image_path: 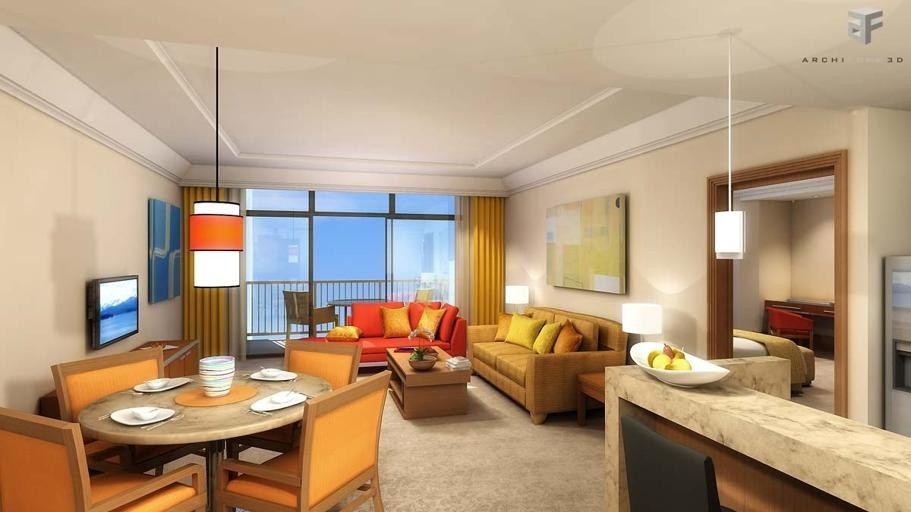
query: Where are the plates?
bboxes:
[133,377,192,392]
[251,370,298,381]
[111,407,175,425]
[250,390,307,412]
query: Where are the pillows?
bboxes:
[326,301,459,342]
[495,308,600,354]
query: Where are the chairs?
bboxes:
[414,288,434,304]
[621,416,737,512]
[225,340,364,512]
[282,290,339,342]
[51,345,210,508]
[767,307,815,351]
[216,369,393,512]
[0,407,207,512]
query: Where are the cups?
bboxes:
[200,355,235,397]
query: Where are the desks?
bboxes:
[327,299,391,326]
[767,298,834,335]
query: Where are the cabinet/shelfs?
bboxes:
[163,339,203,379]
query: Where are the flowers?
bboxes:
[408,327,435,360]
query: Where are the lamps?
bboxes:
[621,303,663,342]
[188,47,244,288]
[286,207,300,264]
[715,32,747,260]
[505,285,529,313]
[420,273,435,289]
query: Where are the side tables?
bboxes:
[576,370,605,426]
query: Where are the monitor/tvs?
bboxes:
[85,276,141,351]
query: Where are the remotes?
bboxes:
[399,346,415,351]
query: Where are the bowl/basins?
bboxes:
[273,393,294,403]
[145,378,169,389]
[129,407,159,419]
[631,340,731,388]
[261,368,281,377]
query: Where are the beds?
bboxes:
[732,336,815,386]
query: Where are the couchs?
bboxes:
[466,306,629,425]
[300,317,467,367]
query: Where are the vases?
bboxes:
[409,355,437,371]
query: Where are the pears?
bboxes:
[648,343,691,371]
